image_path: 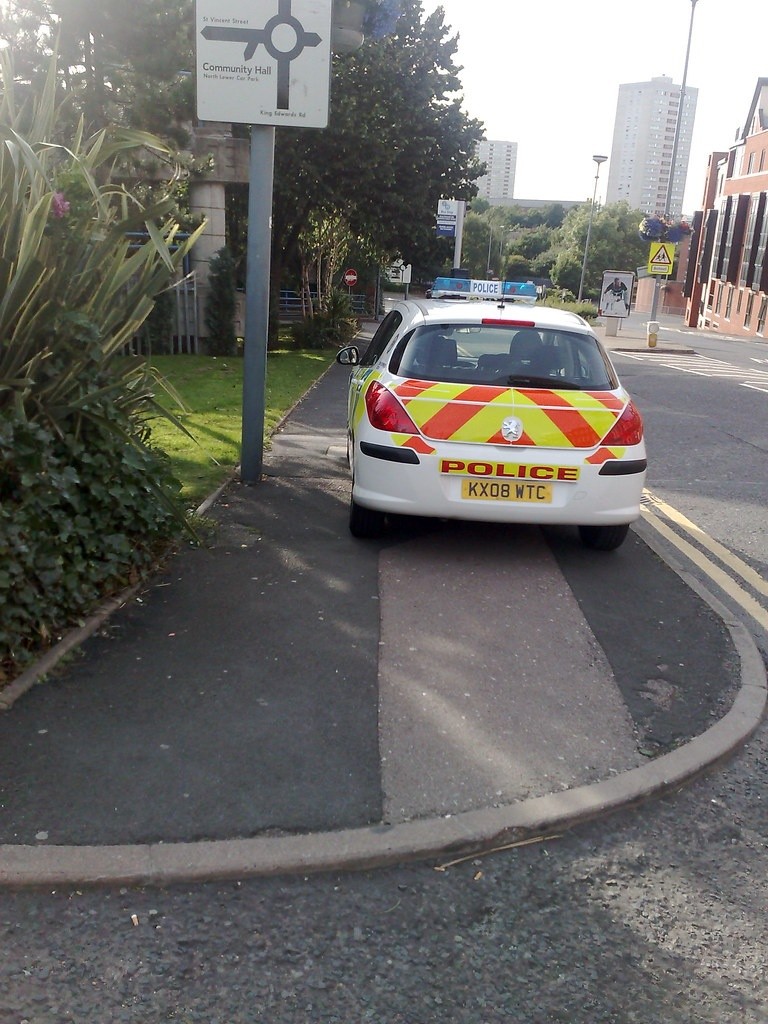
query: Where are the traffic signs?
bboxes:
[196,1,330,130]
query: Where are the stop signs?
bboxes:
[343,268,357,286]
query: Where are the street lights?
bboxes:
[578,155,608,303]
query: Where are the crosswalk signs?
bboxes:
[650,245,671,264]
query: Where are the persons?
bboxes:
[602,277,631,316]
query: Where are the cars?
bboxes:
[336,277,647,549]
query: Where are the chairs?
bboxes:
[417,336,457,367]
[530,345,567,375]
